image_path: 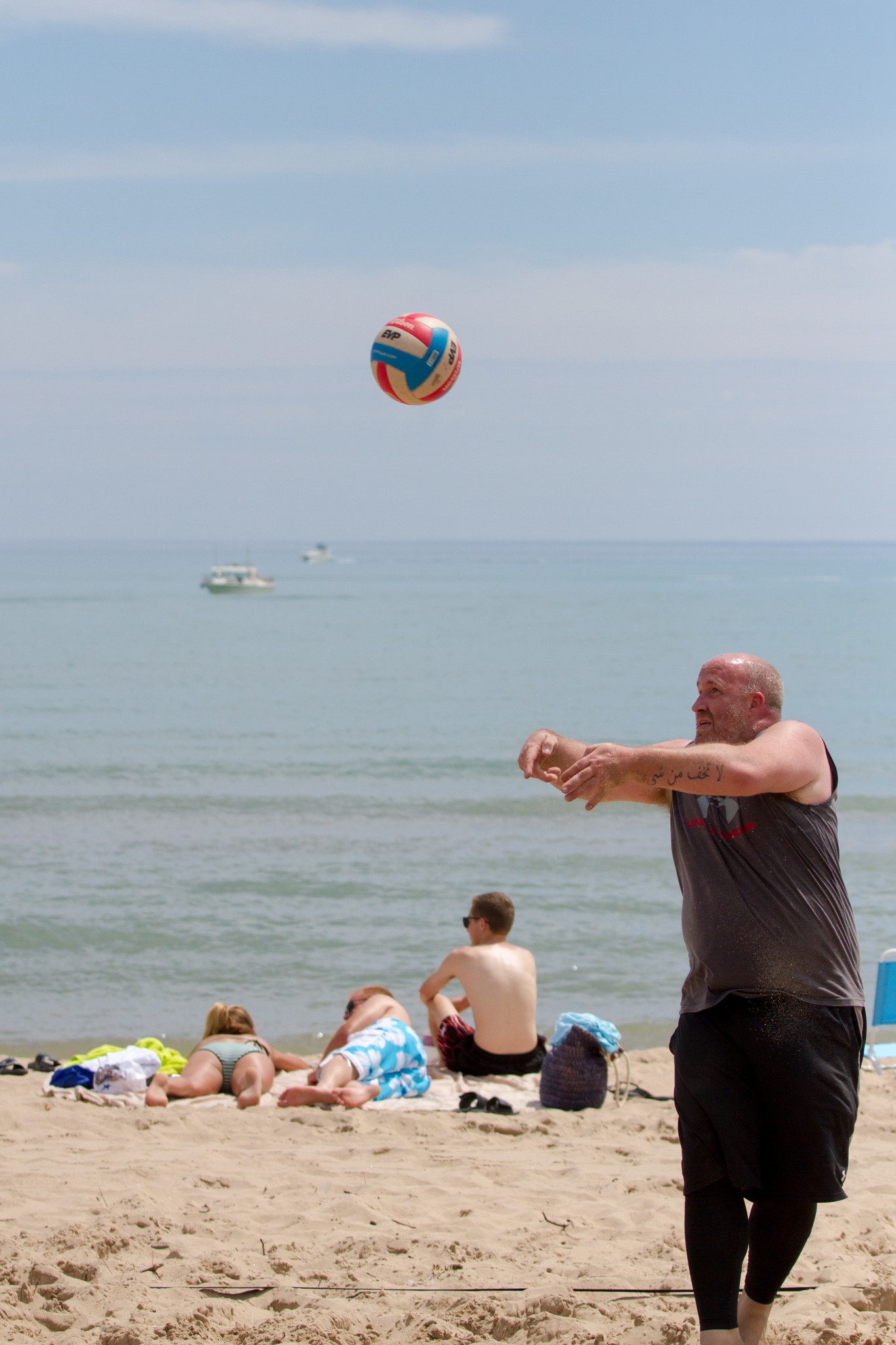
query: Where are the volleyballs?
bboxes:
[371,313,461,405]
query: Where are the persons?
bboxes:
[277,986,432,1110]
[518,652,868,1345]
[420,891,548,1076]
[144,1002,314,1110]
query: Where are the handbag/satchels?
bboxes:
[539,1021,631,1110]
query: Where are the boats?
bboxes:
[200,564,278,594]
[302,537,333,562]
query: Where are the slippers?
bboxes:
[28,1053,61,1072]
[0,1057,27,1074]
[452,1091,520,1116]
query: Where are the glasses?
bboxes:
[344,998,368,1015]
[462,917,490,928]
